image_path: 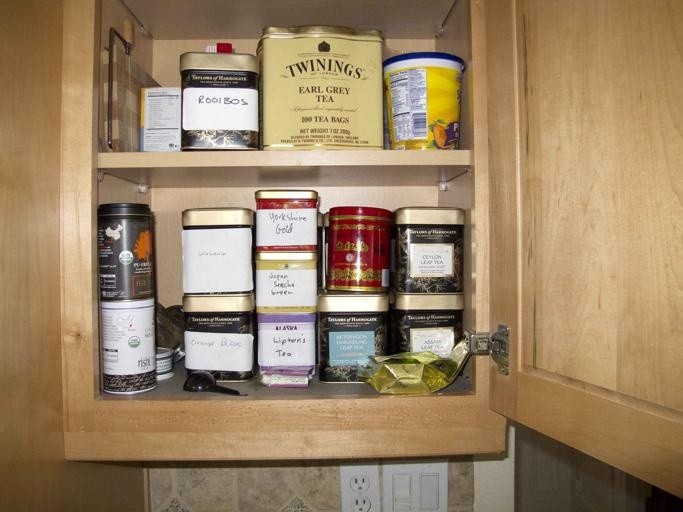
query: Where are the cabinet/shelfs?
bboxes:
[60,1,683,501]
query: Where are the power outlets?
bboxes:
[339,465,381,512]
